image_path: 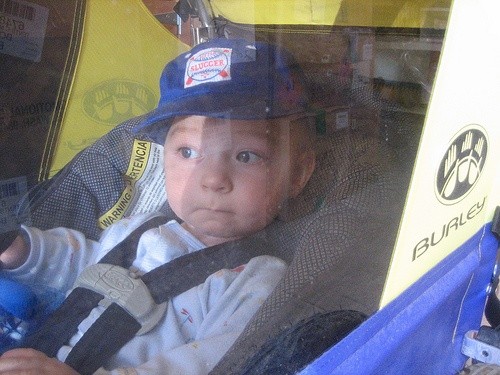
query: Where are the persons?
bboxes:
[0,37,316,375]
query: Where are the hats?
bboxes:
[133,38,316,137]
